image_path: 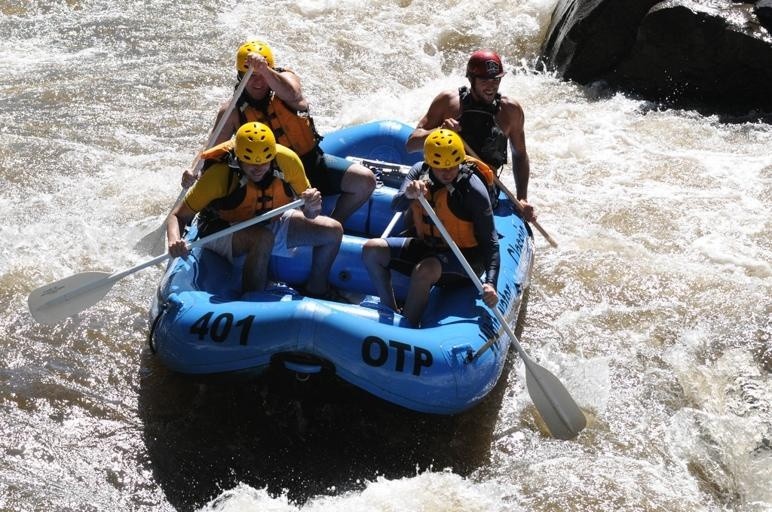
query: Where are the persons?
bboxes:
[180,41,376,223]
[167,121,351,303]
[361,126,500,326]
[405,51,533,217]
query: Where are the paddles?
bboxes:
[29,188,318,328]
[417,192,587,440]
[134,63,254,257]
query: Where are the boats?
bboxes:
[148,119,534,419]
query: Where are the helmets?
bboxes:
[423,129,467,169]
[466,50,507,80]
[234,122,277,165]
[235,41,275,73]
